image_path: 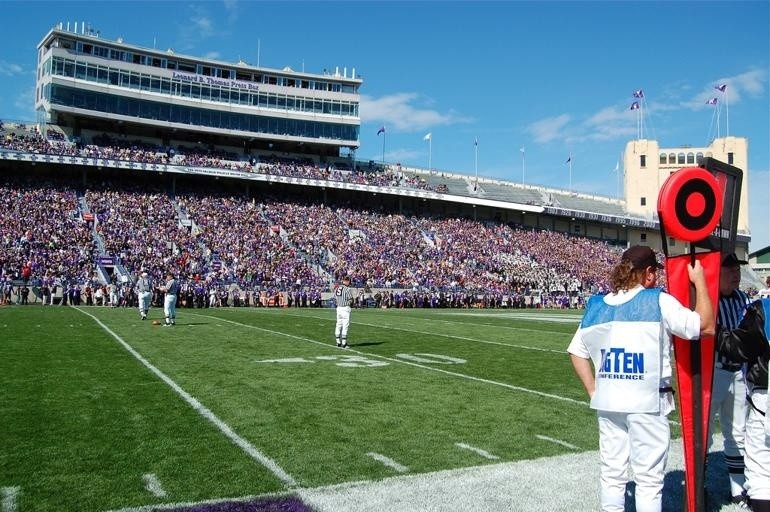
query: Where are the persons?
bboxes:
[0,124,768,310]
[159,272,179,327]
[134,272,154,320]
[566,246,716,512]
[716,298,770,512]
[333,277,354,349]
[703,253,753,510]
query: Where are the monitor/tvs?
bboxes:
[695,156,743,253]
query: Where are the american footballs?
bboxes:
[151,319,162,325]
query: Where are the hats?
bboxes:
[722,253,748,267]
[622,245,664,269]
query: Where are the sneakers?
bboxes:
[141,314,146,320]
[729,490,751,512]
[163,321,175,327]
[336,343,351,348]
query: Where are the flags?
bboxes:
[630,89,643,109]
[705,97,717,104]
[715,85,726,92]
